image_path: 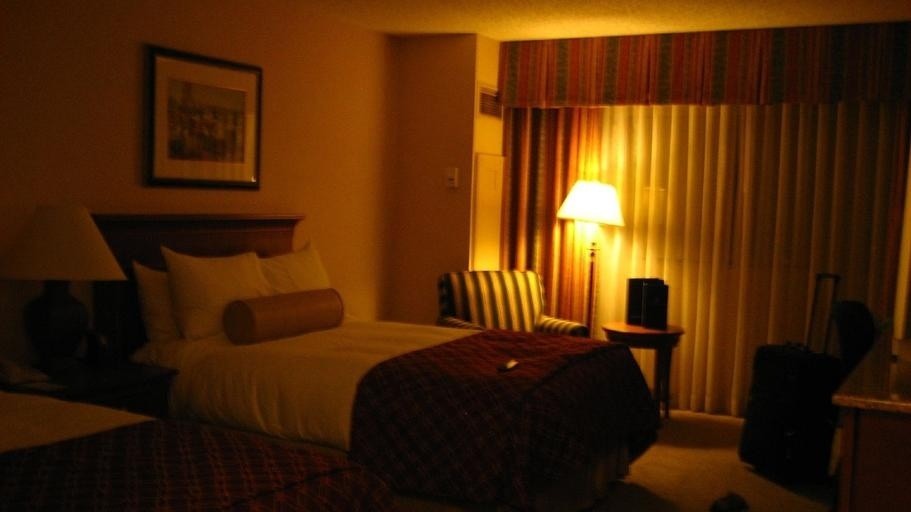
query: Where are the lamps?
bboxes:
[557,180,625,328]
[0,204,127,371]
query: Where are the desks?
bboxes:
[833,322,911,512]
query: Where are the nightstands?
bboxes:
[0,363,181,416]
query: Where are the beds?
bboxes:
[90,213,658,512]
[1,392,390,512]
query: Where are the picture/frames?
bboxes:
[140,43,263,191]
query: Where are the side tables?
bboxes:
[601,321,684,418]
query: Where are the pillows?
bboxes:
[161,246,272,342]
[223,288,344,345]
[134,261,181,341]
[259,237,331,293]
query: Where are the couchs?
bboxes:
[436,270,590,338]
